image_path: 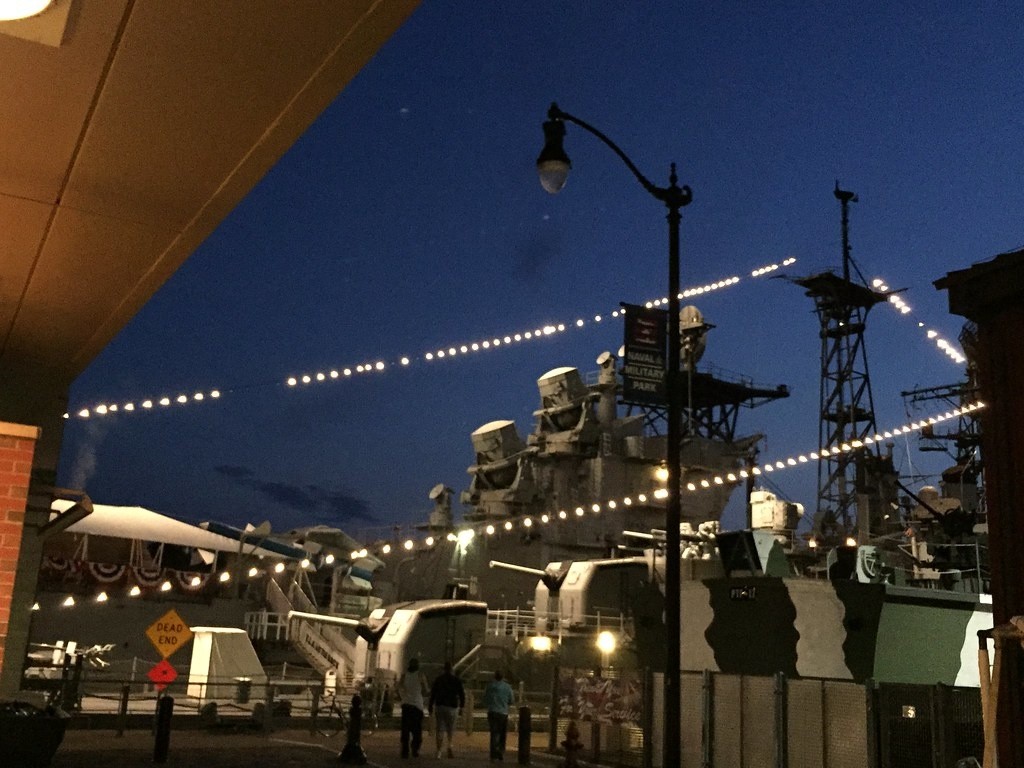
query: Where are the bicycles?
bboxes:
[312,689,380,740]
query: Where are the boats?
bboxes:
[18,499,401,697]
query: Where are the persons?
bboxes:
[429,661,465,759]
[398,658,430,759]
[483,670,515,763]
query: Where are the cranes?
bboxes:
[901,365,980,464]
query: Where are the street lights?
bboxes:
[535,95,699,768]
[530,633,563,756]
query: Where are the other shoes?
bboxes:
[490,754,497,760]
[436,752,440,759]
[400,751,408,759]
[448,746,455,758]
[499,756,503,760]
[412,752,421,757]
